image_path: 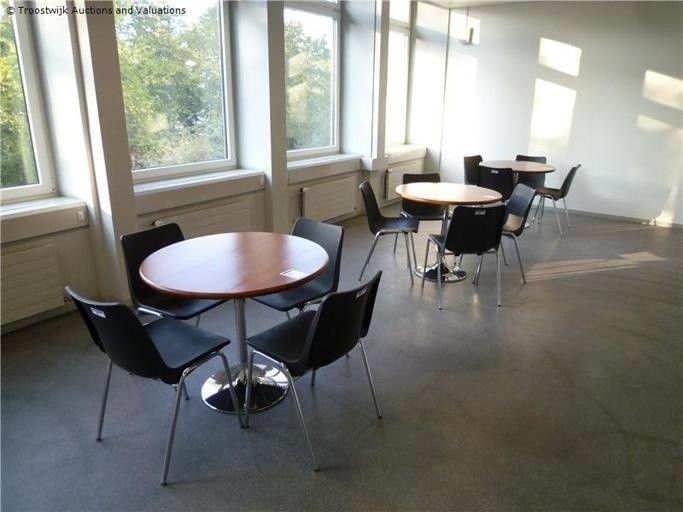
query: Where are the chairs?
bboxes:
[254,218,345,321]
[243,270,383,471]
[66,286,246,485]
[359,153,581,309]
[120,222,237,328]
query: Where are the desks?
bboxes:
[137,232,330,414]
